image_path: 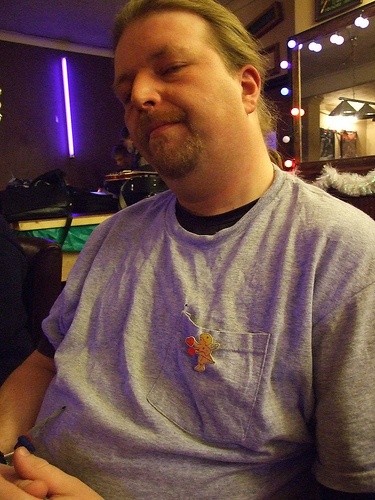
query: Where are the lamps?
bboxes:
[329,34,375,119]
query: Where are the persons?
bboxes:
[97,126,168,211]
[0,0,375,500]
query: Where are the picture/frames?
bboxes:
[244,2,283,38]
[315,0,363,21]
[258,42,281,78]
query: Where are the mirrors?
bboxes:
[287,2,375,176]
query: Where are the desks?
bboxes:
[8,211,119,282]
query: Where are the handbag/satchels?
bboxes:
[7,168,68,207]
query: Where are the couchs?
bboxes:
[15,236,61,346]
